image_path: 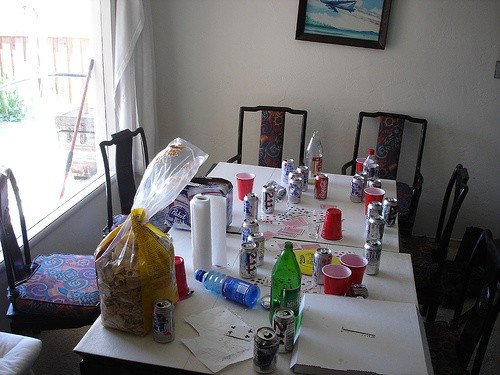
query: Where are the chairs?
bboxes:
[0,106,500,375]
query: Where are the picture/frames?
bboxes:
[294,0,393,50]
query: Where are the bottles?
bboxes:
[193,268,260,308]
[363,149,379,188]
[268,241,302,337]
[305,130,323,185]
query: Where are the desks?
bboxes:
[171,162,401,253]
[73,227,419,375]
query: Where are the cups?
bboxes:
[363,186,386,216]
[175,256,189,301]
[356,158,374,172]
[321,263,352,296]
[339,253,368,286]
[235,172,256,201]
[320,208,343,241]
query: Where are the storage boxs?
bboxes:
[290,293,434,375]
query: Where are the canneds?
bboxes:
[296,165,309,191]
[350,172,382,203]
[364,239,382,275]
[152,299,175,343]
[315,174,328,199]
[281,158,295,184]
[367,201,383,217]
[365,215,385,246]
[242,193,258,220]
[346,285,369,300]
[271,308,296,353]
[382,197,398,226]
[239,218,265,278]
[252,327,280,374]
[262,171,302,213]
[313,248,333,285]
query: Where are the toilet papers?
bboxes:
[189,193,227,272]
